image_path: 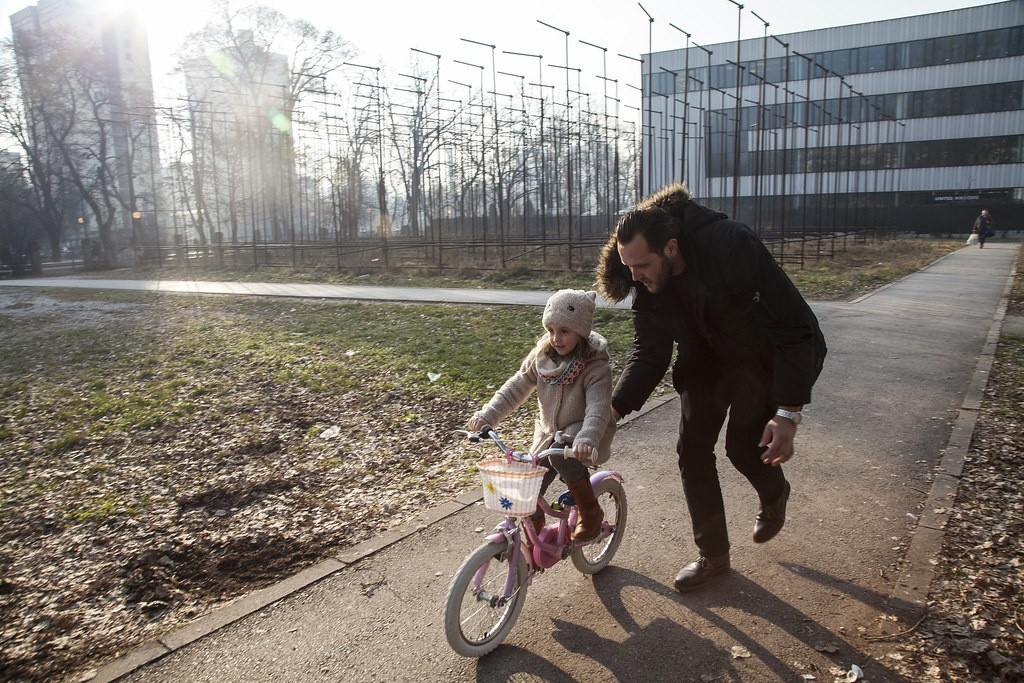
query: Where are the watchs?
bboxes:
[776,408,802,425]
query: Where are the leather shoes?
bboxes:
[752,479,791,544]
[672,553,730,590]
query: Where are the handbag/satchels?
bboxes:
[983,231,996,237]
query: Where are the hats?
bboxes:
[542,289,596,340]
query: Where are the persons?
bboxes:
[972,208,993,249]
[594,185,828,593]
[468,288,617,572]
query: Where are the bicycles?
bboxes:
[439,417,630,660]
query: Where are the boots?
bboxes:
[564,470,604,542]
[520,517,545,550]
